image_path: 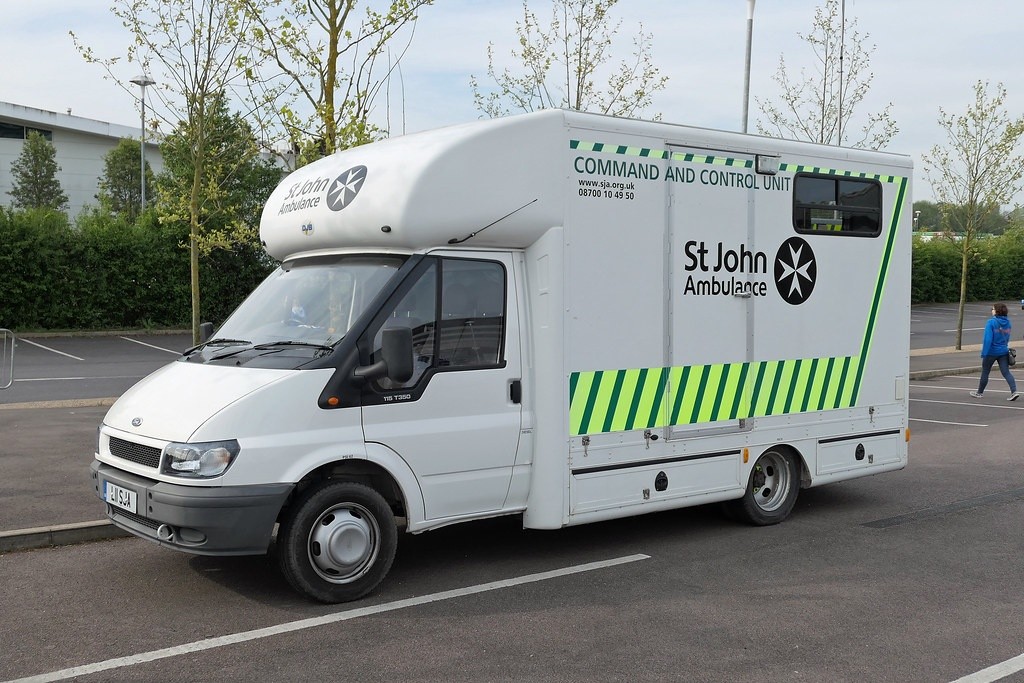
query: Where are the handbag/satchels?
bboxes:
[1008,347,1016,366]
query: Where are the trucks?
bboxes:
[91,108,913,606]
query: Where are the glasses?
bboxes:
[992,308,995,310]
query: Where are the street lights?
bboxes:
[128,75,157,212]
[915,211,922,230]
[914,218,917,230]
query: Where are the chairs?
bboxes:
[416,283,480,364]
[385,289,423,346]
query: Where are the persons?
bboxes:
[970,302,1019,401]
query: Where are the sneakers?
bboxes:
[1006,391,1019,401]
[970,391,984,398]
